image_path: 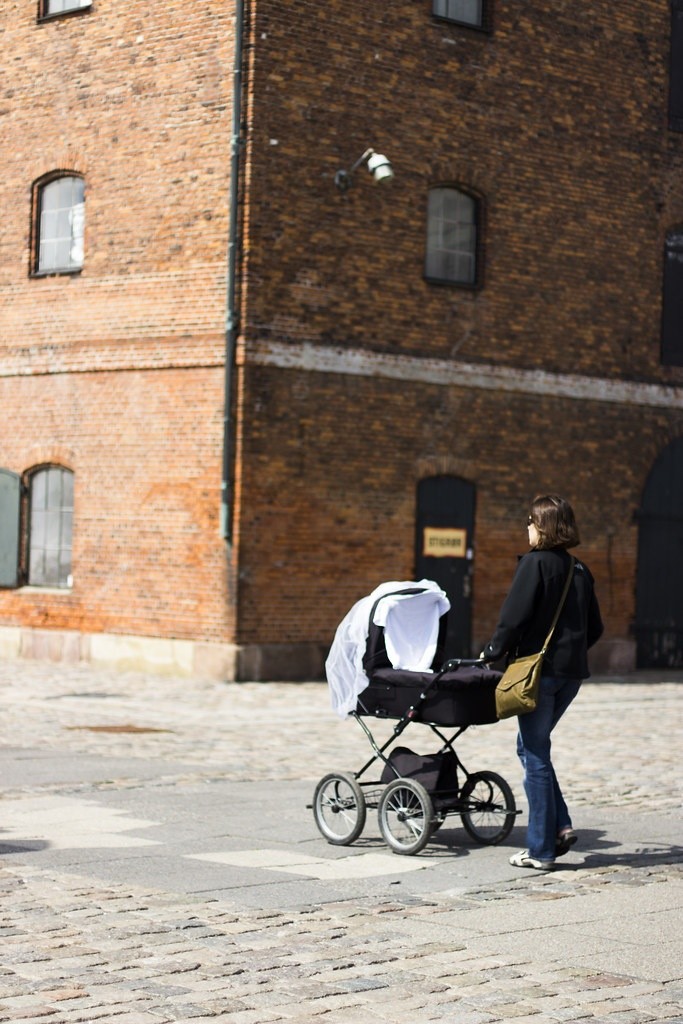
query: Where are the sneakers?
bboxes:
[508,848,554,870]
[552,823,579,857]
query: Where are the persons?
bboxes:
[480,496,604,868]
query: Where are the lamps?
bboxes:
[334,147,395,194]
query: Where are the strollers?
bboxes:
[311,578,523,855]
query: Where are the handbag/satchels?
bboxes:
[381,745,458,799]
[494,650,543,717]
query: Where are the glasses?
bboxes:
[526,515,534,523]
